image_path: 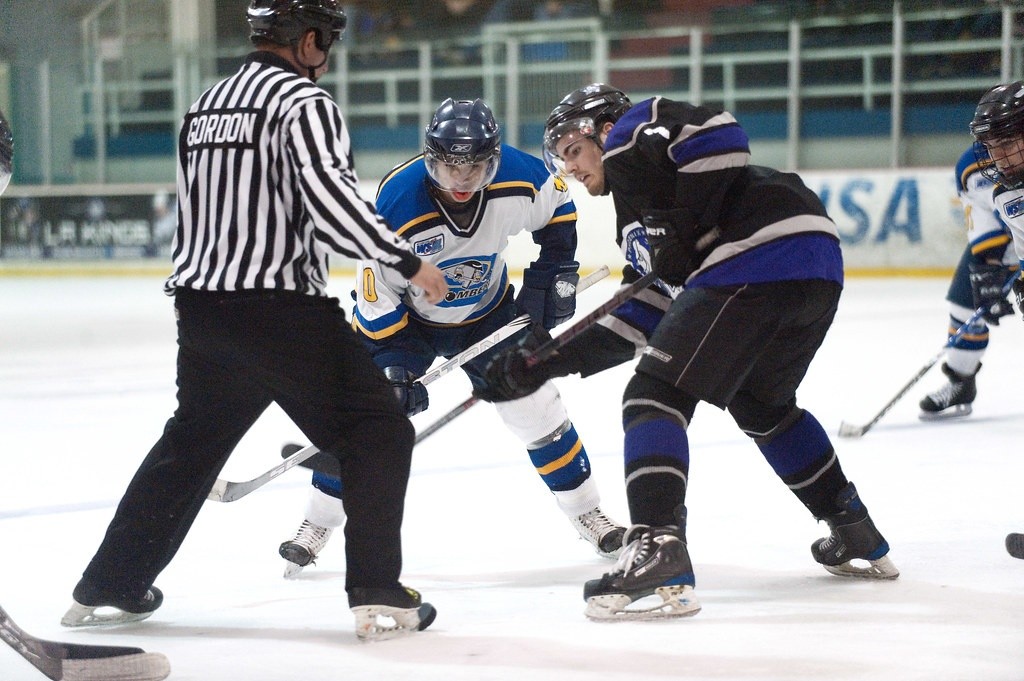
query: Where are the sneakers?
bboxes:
[573,506,630,558]
[348,588,436,638]
[812,481,900,578]
[279,520,332,577]
[60,578,164,626]
[584,504,701,618]
[917,363,982,419]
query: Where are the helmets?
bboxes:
[245,0,346,52]
[424,98,501,164]
[545,84,633,138]
[969,81,1024,142]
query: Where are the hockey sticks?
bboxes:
[203,263,614,503]
[1004,532,1024,560]
[281,269,661,474]
[0,604,172,681]
[836,269,1024,440]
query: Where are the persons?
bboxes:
[919,81,1024,421]
[59,0,436,640]
[478,82,900,621]
[339,0,1024,108]
[279,98,628,580]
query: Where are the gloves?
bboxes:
[485,323,568,401]
[641,208,699,286]
[513,261,580,330]
[384,366,429,418]
[967,260,1014,325]
[1012,278,1024,313]
